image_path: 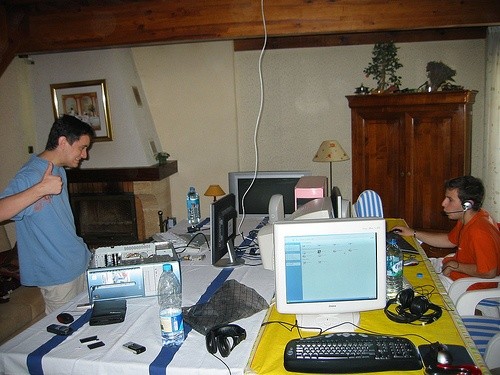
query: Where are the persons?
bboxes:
[392,175,500,315]
[0,115,94,314]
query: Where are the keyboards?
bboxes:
[284,333,422,374]
[153,231,197,250]
[384,232,419,254]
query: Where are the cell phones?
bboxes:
[47,324,73,336]
[122,341,146,354]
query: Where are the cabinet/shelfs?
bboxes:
[346,93,478,257]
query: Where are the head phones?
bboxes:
[382,288,443,326]
[206,324,246,357]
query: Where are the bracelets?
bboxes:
[414,230,416,238]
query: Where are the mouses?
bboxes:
[57,313,75,324]
[403,258,419,266]
[429,341,452,367]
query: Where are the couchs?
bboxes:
[0,220,49,344]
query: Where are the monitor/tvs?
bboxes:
[272,217,386,333]
[210,193,245,268]
[228,171,305,218]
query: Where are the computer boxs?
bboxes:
[294,176,328,211]
[85,242,182,304]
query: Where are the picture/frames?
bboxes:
[50,79,112,142]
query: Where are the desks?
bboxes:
[0,215,493,375]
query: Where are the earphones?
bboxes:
[463,202,472,210]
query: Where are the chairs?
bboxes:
[444,253,500,305]
[456,289,500,369]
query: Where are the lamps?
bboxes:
[204,185,226,202]
[312,140,350,196]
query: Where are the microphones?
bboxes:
[440,209,466,216]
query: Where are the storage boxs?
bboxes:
[87,242,181,303]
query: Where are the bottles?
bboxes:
[157,264,185,346]
[187,187,200,225]
[386,238,403,302]
